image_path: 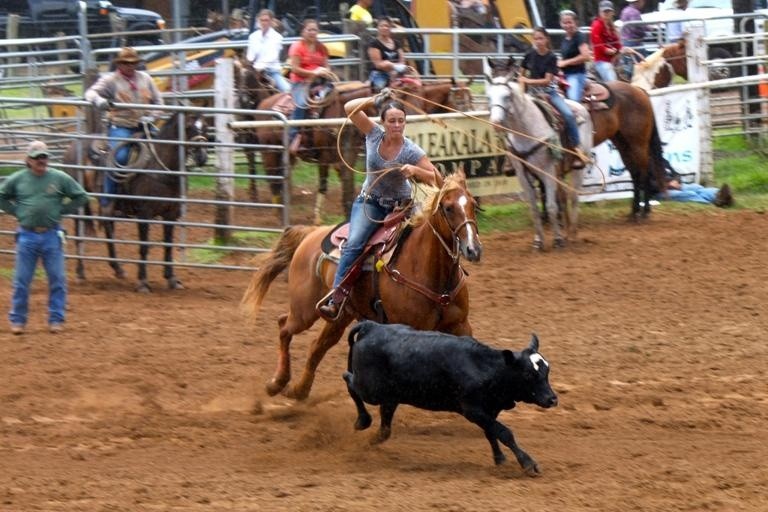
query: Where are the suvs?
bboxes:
[616,1,768,87]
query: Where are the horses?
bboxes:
[240,163,483,401]
[630,38,687,90]
[231,53,274,204]
[482,72,595,254]
[256,74,423,226]
[341,77,475,114]
[64,110,208,295]
[487,55,670,227]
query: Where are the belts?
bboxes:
[20,225,50,233]
[361,191,411,210]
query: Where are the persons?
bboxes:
[319,88,437,315]
[83,44,168,227]
[521,27,595,167]
[239,1,691,156]
[1,137,93,339]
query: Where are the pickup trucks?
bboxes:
[0,0,166,81]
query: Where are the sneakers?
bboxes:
[11,323,65,334]
[570,156,585,169]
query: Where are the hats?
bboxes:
[600,1,613,12]
[27,141,52,160]
[112,47,142,64]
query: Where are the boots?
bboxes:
[320,299,344,320]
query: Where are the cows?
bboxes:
[342,319,558,478]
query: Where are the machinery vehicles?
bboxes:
[39,1,563,153]
